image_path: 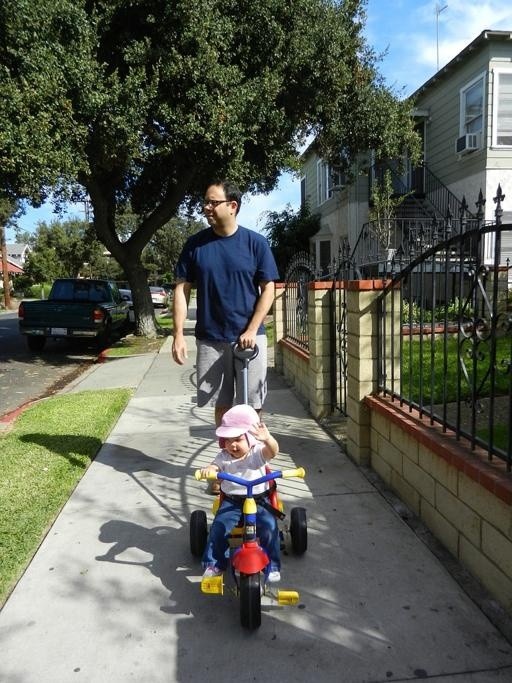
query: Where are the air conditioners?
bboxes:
[455,133,478,156]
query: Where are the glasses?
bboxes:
[203,200,231,206]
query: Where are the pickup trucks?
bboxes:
[17,277,131,357]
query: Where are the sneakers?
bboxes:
[268,571,281,583]
[203,566,219,578]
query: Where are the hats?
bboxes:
[215,404,260,447]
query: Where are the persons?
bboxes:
[198,404,286,581]
[171,179,278,443]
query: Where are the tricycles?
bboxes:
[189,465,308,635]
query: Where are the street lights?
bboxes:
[435,1,449,71]
[83,262,91,278]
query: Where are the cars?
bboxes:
[118,288,133,307]
[149,286,170,308]
